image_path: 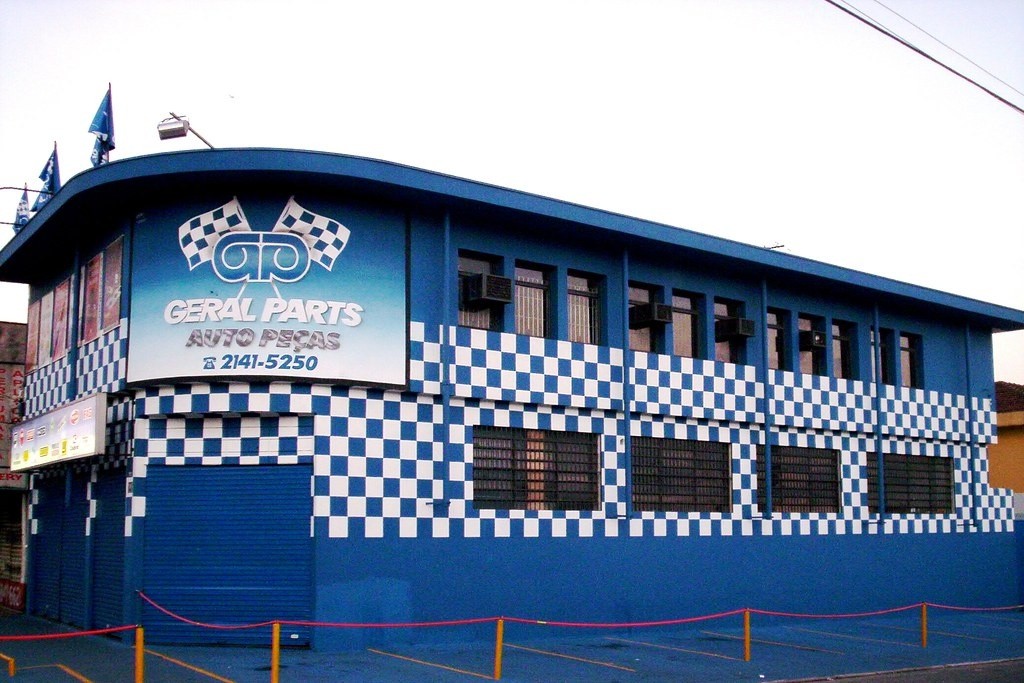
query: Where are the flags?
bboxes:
[30,149,61,213]
[88,89,116,165]
[13,187,30,235]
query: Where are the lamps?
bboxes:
[157,120,189,140]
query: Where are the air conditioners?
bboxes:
[463,273,513,307]
[801,330,827,351]
[637,303,672,324]
[725,317,755,337]
